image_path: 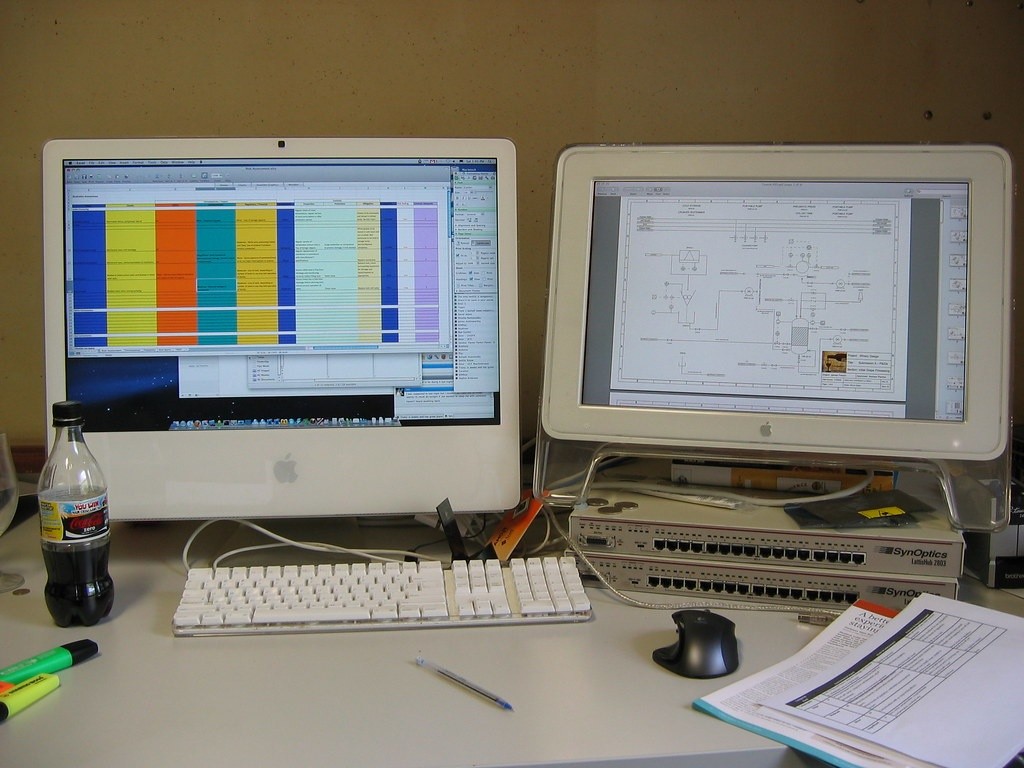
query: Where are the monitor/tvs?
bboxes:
[534,146,1013,527]
[38,137,520,565]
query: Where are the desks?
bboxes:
[0,473,1024,768]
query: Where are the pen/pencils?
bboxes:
[413,653,515,713]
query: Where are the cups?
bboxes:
[0,433,20,536]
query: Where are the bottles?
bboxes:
[34,399,116,628]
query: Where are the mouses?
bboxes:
[650,609,738,680]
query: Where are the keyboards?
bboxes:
[171,556,592,636]
